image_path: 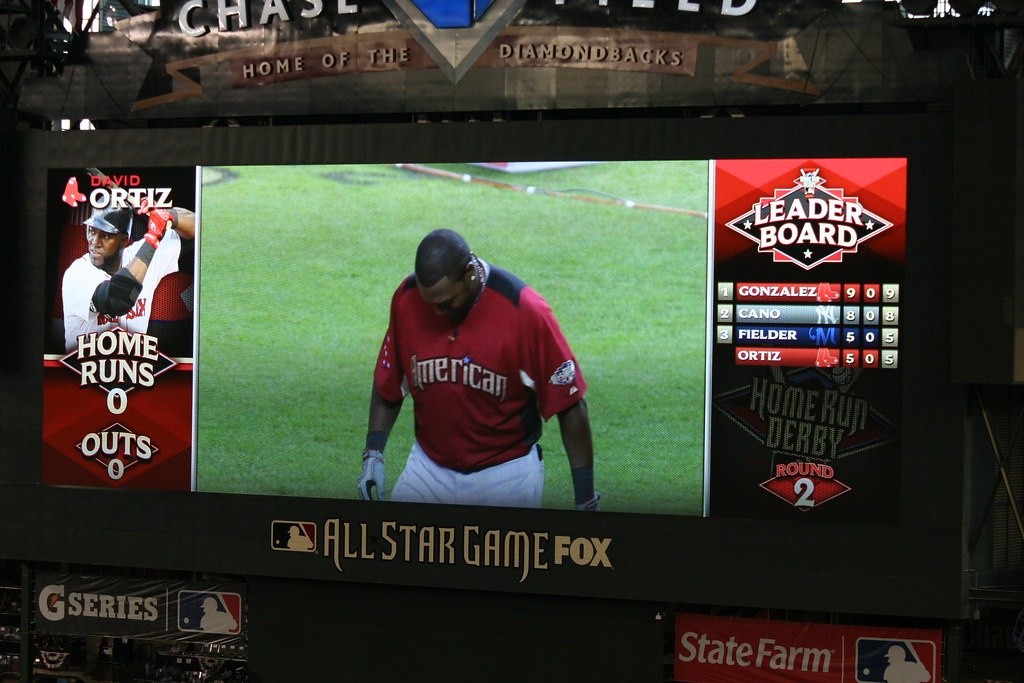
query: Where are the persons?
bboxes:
[62,195,196,355]
[357,229,601,511]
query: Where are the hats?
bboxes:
[83,193,133,234]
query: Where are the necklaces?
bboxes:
[472,254,485,301]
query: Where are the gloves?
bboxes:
[357,446,386,500]
[134,196,162,214]
[576,492,601,511]
[144,208,174,248]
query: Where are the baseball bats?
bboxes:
[87,168,172,230]
